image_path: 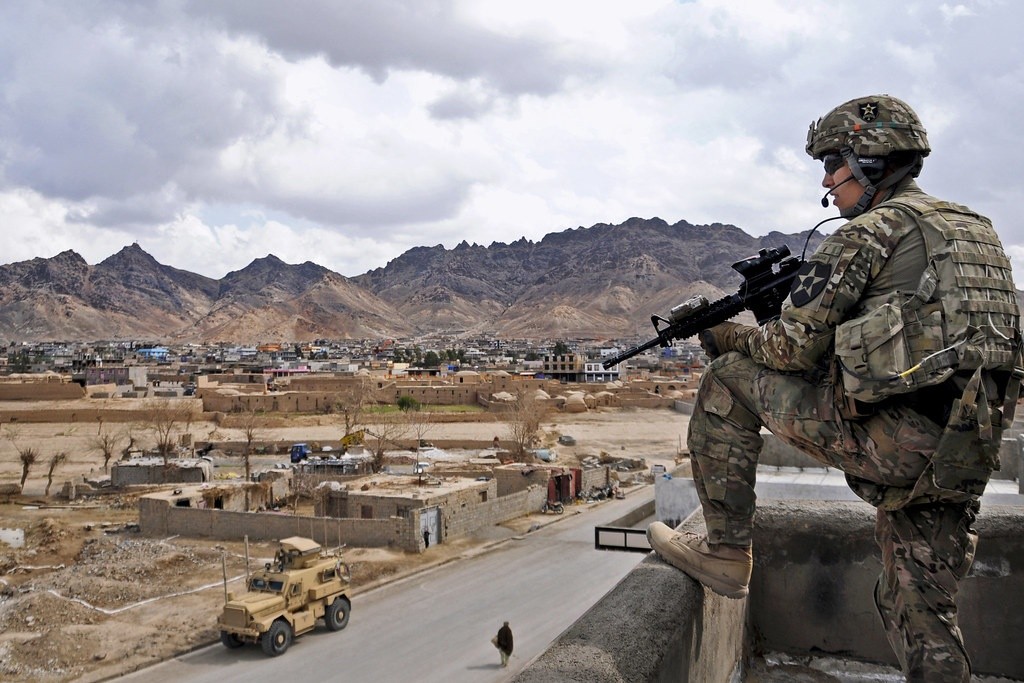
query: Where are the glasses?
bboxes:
[823,150,845,174]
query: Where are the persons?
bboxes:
[494,620,513,667]
[650,93,1024,683]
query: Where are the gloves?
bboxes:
[698,320,743,361]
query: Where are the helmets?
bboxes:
[807,97,931,159]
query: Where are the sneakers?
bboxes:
[645,522,753,599]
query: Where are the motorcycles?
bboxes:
[540,498,565,515]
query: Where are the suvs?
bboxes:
[217,536,353,657]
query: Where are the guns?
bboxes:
[600,243,809,371]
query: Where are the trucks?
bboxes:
[290,443,345,464]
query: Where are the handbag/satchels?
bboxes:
[834,303,916,402]
